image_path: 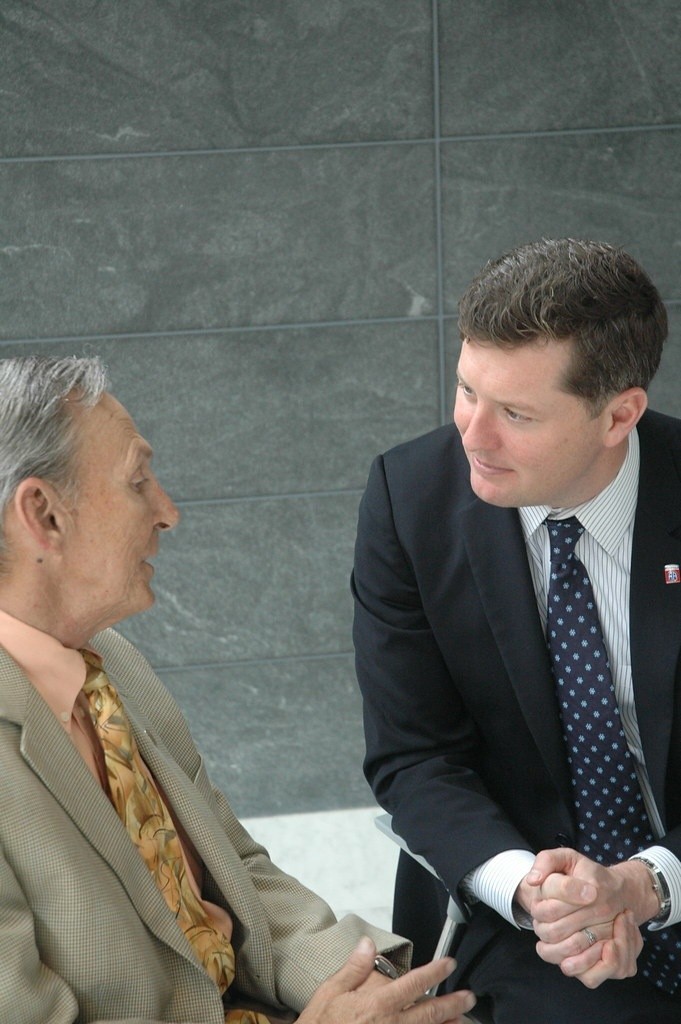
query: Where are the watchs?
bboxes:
[374,955,399,978]
[632,857,672,920]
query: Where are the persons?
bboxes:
[0,356,474,1024]
[350,239,681,1024]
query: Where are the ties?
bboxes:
[542,517,681,996]
[77,651,270,1024]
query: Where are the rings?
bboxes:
[583,929,596,945]
